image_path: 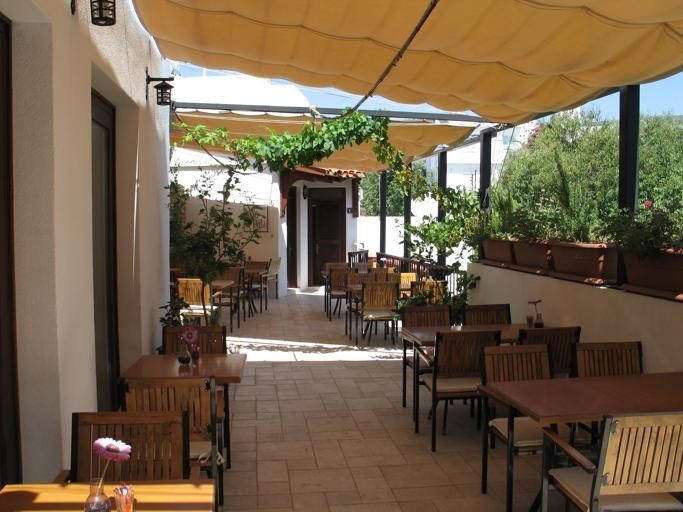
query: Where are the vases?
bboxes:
[84,477,112,512]
[533,313,544,327]
[622,248,683,294]
[178,342,190,363]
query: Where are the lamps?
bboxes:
[71,0,116,28]
[145,66,174,106]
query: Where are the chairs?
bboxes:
[118,374,222,504]
[71,410,190,480]
[462,303,511,325]
[518,325,581,383]
[411,281,447,304]
[478,343,594,512]
[571,342,643,453]
[423,329,501,452]
[314,245,417,345]
[172,256,283,330]
[402,305,450,327]
[541,412,683,510]
[161,325,226,355]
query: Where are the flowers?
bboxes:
[609,201,683,248]
[528,298,543,316]
[91,436,131,500]
[182,327,196,349]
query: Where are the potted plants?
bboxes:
[512,193,550,270]
[549,198,608,278]
[482,186,513,267]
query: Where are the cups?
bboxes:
[526,315,535,330]
[115,489,134,512]
[190,346,201,368]
